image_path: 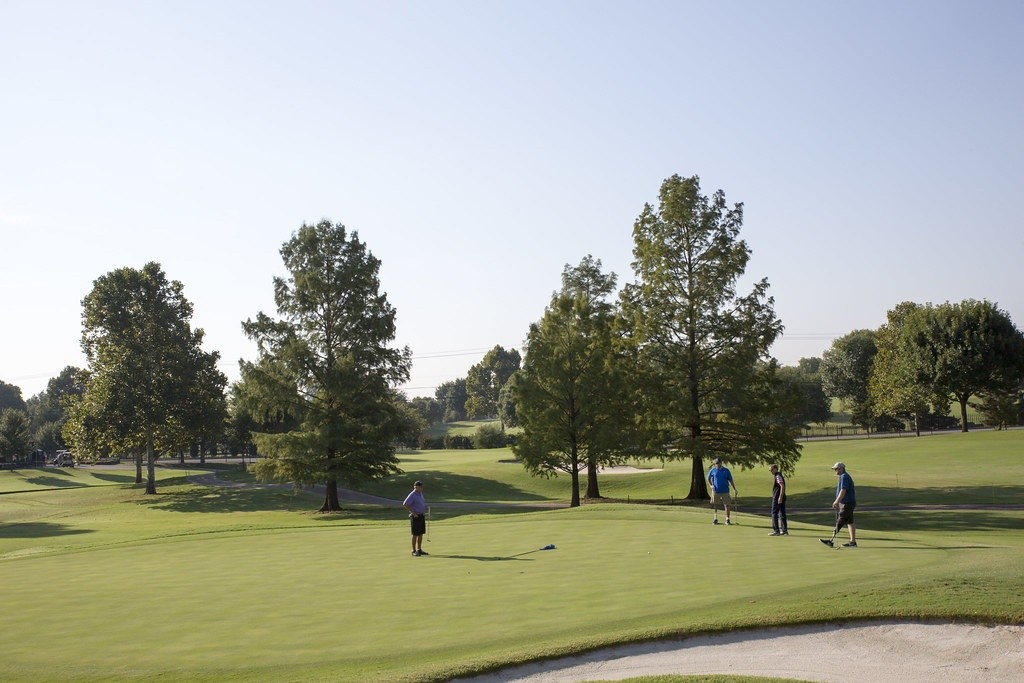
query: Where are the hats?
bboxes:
[831,462,846,469]
[715,457,722,464]
[413,481,423,486]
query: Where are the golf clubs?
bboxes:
[735,493,739,525]
[835,509,840,550]
[427,506,432,543]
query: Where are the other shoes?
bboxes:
[780,531,789,535]
[768,531,780,536]
[725,519,730,525]
[416,550,428,555]
[713,519,718,524]
[412,551,421,556]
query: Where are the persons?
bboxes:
[707,458,738,525]
[768,465,788,536]
[403,481,431,556]
[819,463,857,548]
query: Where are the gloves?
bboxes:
[733,489,738,495]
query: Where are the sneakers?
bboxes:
[842,541,857,547]
[819,538,833,547]
